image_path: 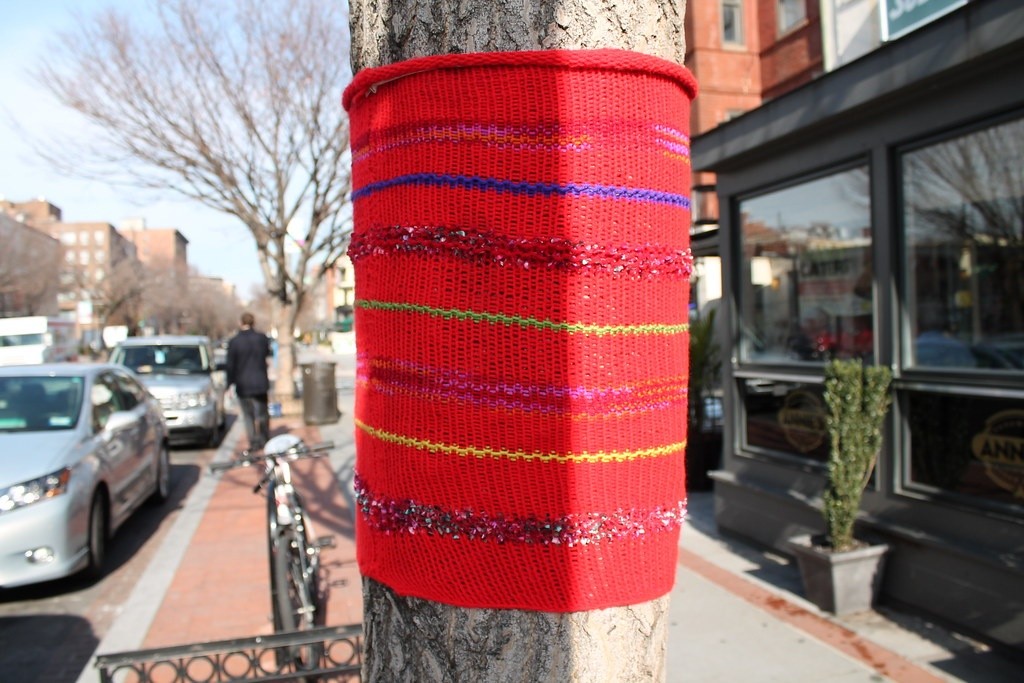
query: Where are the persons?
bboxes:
[225,313,274,448]
[916,302,975,367]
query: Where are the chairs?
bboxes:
[52,387,79,414]
[18,385,51,412]
[134,349,155,366]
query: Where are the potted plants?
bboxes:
[786,357,890,617]
[683,307,723,496]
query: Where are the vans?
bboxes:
[0,316,79,365]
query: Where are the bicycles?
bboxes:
[209,435,339,683]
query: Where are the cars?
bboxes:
[742,318,1024,506]
[0,364,170,588]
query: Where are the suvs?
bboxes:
[109,335,226,448]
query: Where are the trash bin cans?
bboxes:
[301,362,339,425]
